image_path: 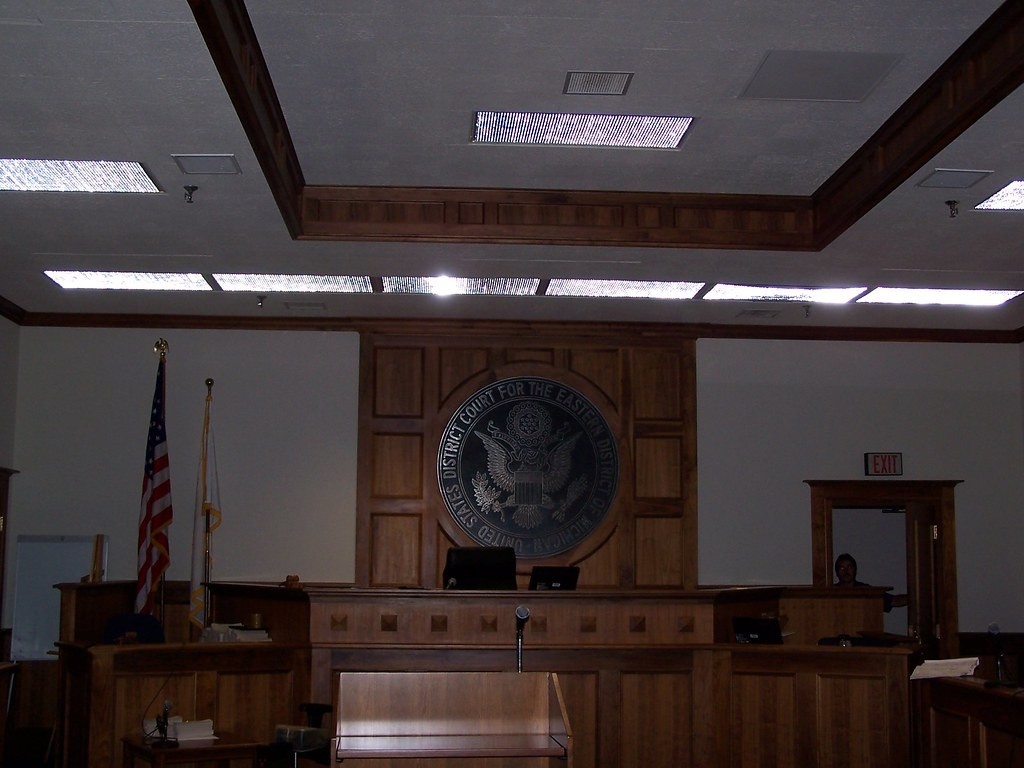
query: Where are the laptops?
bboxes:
[528,566,580,591]
[729,616,784,646]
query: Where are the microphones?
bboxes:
[516,606,530,634]
[445,578,456,589]
[988,622,1005,660]
[162,703,171,732]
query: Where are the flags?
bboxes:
[187,395,222,634]
[134,359,172,618]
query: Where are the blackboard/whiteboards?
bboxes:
[10,532,110,662]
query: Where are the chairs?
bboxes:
[443,547,521,592]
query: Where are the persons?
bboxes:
[832,554,908,622]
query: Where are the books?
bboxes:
[909,655,980,681]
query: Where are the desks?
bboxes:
[122,728,264,768]
